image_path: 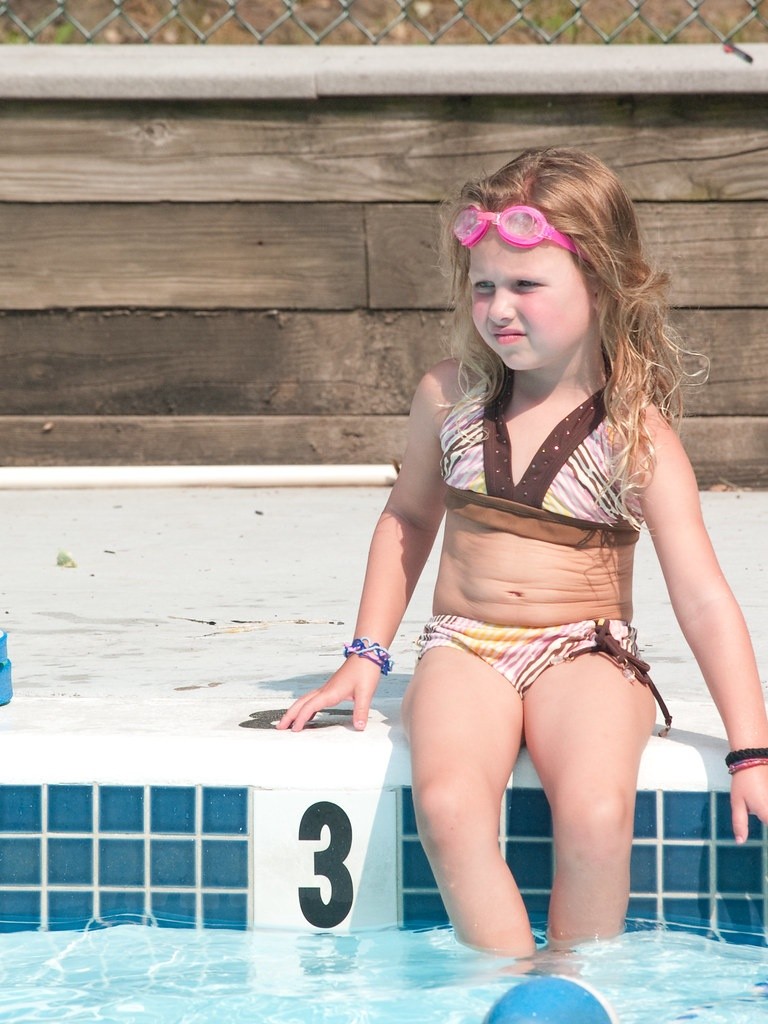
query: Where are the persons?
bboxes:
[275,143,768,938]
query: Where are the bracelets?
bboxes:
[343,637,394,675]
[725,748,768,774]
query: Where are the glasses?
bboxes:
[453,203,584,253]
